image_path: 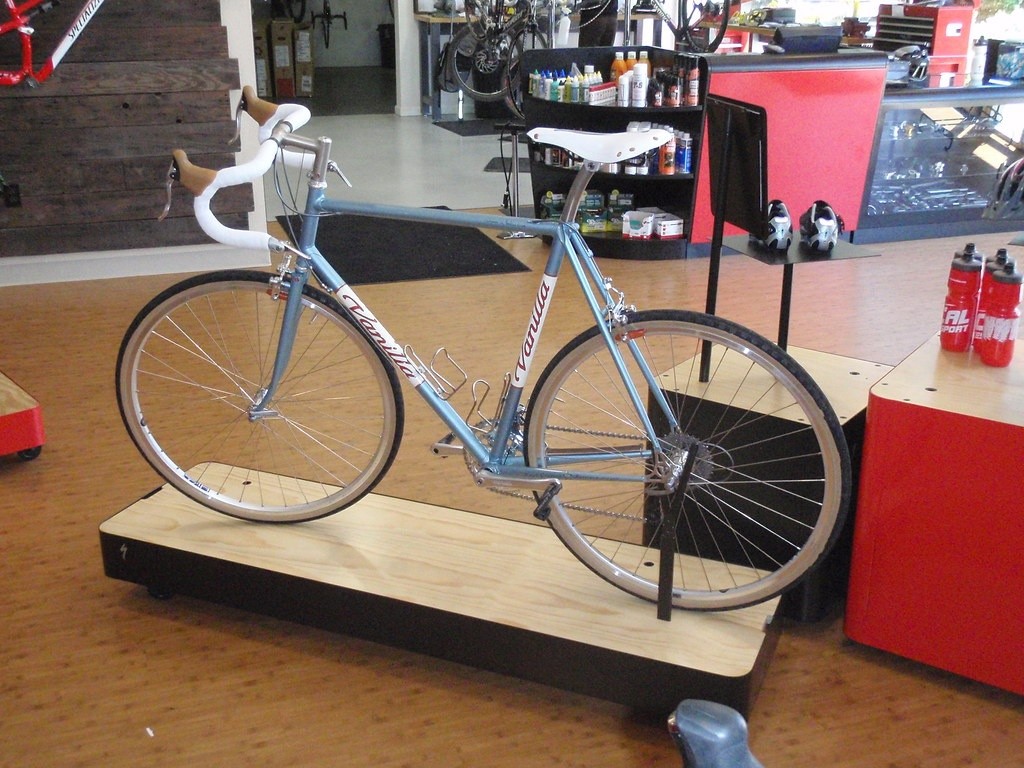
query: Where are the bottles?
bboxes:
[940,242,1023,367]
[610,50,651,88]
[627,122,692,176]
[652,53,701,108]
[531,68,606,102]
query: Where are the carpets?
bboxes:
[433,118,532,136]
[484,157,533,173]
[274,206,533,288]
[497,132,530,144]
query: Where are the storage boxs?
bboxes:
[621,206,685,240]
[252,14,315,98]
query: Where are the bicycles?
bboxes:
[115,83,850,623]
[448,1,550,120]
[556,0,732,53]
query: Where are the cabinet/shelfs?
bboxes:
[518,45,713,261]
[849,77,1024,244]
